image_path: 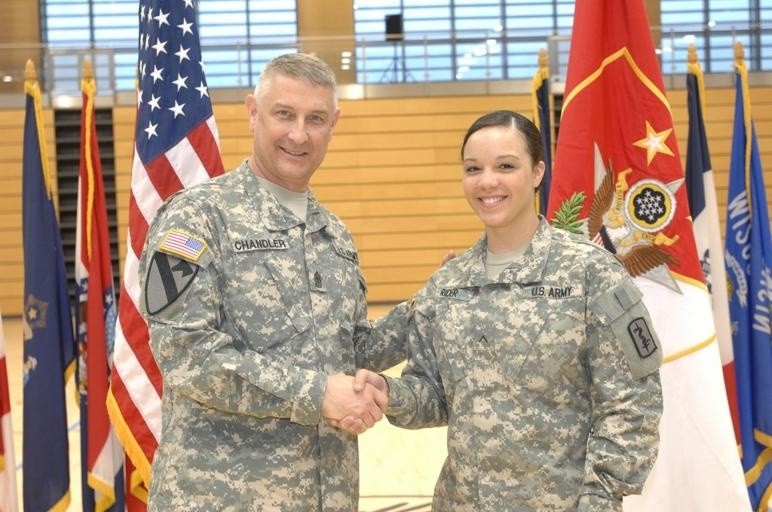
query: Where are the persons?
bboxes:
[135,52,458,511]
[326,109,665,512]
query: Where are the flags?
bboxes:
[528,52,557,219]
[104,0,224,511]
[547,2,754,512]
[74,77,127,512]
[681,48,744,471]
[22,79,77,512]
[724,49,771,511]
